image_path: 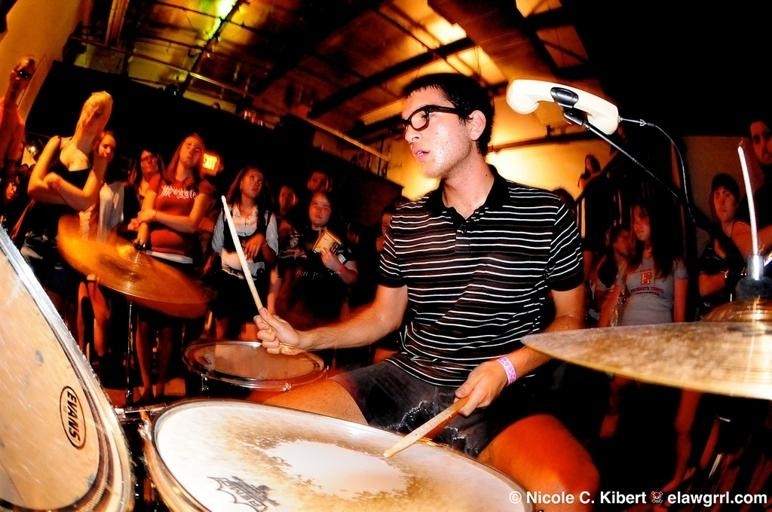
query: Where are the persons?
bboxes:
[254,72,601,511]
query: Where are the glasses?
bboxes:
[385,103,464,141]
[751,129,771,145]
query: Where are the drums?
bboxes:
[143,399,535,512]
[0,225,133,512]
[183,339,330,395]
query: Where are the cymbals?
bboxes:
[519,299,772,400]
[52,220,211,320]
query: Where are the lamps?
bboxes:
[506,75,702,321]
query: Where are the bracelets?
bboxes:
[497,355,517,386]
